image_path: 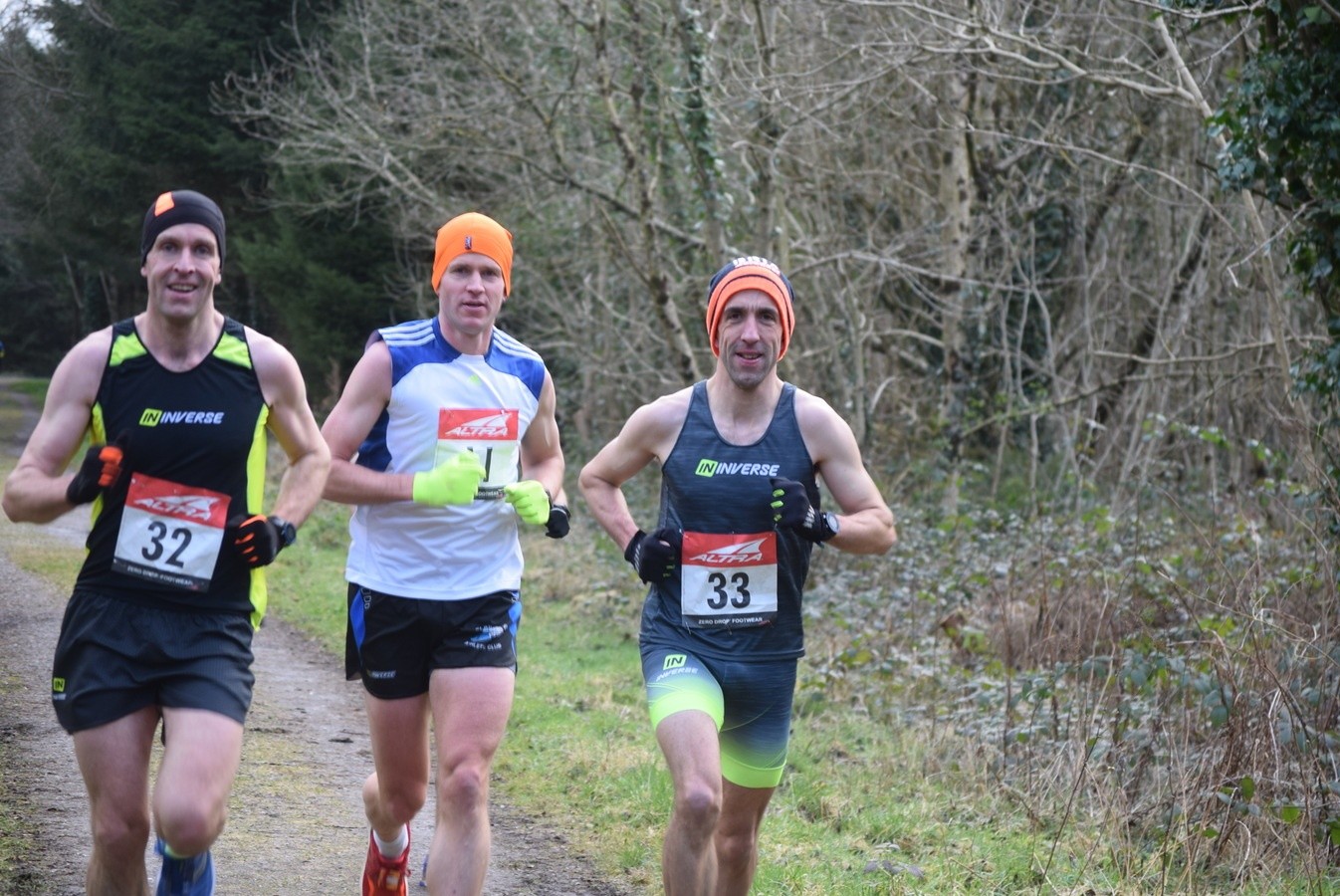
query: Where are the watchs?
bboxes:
[268,515,296,547]
[814,510,839,548]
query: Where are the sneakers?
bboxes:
[361,820,412,896]
[152,830,215,896]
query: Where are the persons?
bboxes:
[318,210,570,896]
[0,188,332,896]
[576,257,897,896]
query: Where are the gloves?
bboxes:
[227,513,287,569]
[413,449,487,507]
[67,443,123,503]
[504,480,549,526]
[623,524,682,585]
[769,477,836,543]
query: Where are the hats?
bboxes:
[431,211,514,302]
[140,192,224,271]
[706,256,795,363]
